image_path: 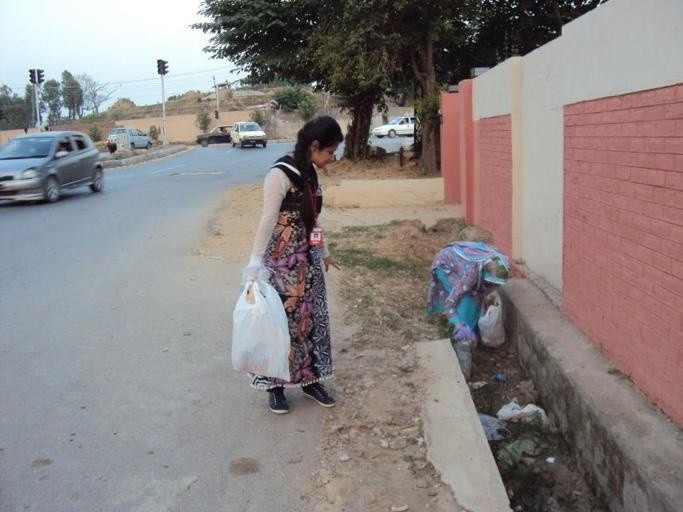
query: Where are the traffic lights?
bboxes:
[157,59,169,74]
[29,69,36,83]
[37,69,45,84]
[215,110,218,119]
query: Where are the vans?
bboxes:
[0,130,104,203]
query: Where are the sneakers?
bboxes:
[301,382,336,408]
[266,387,289,414]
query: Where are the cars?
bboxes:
[195,121,267,148]
[373,116,414,138]
[106,127,152,153]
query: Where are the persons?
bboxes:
[57,143,66,151]
[45,127,49,131]
[23,128,29,135]
[428,238,513,348]
[244,117,342,413]
